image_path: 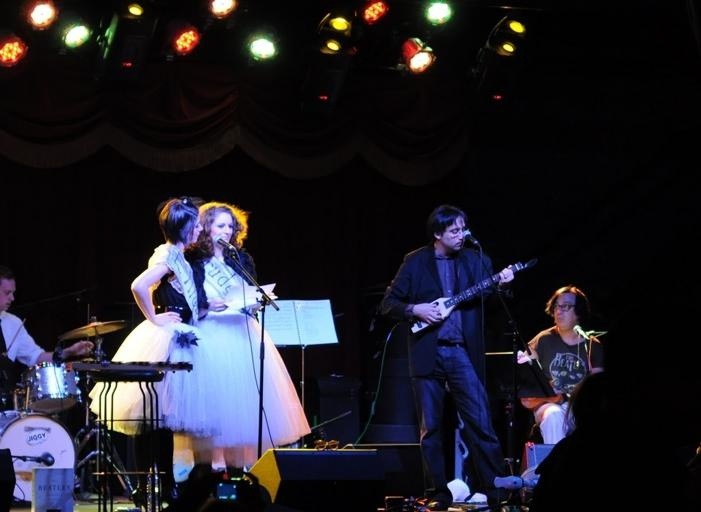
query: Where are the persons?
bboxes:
[380,203,517,510]
[1,270,94,414]
[520,286,607,446]
[188,202,315,476]
[88,199,224,438]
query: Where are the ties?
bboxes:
[0,319,6,354]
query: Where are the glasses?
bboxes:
[552,303,576,312]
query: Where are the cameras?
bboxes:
[213,481,250,503]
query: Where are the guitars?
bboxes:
[407,259,529,335]
[520,385,568,411]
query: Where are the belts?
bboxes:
[438,338,465,348]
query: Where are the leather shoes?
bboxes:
[428,498,448,511]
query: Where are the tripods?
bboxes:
[74,331,134,500]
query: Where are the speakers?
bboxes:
[518,444,557,500]
[251,448,421,504]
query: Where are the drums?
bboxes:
[0,410,76,502]
[21,362,81,411]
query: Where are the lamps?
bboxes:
[301,1,365,55]
[400,38,436,75]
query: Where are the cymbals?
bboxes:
[58,320,127,342]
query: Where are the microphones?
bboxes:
[572,325,590,340]
[212,234,233,250]
[461,230,483,249]
[41,453,53,466]
